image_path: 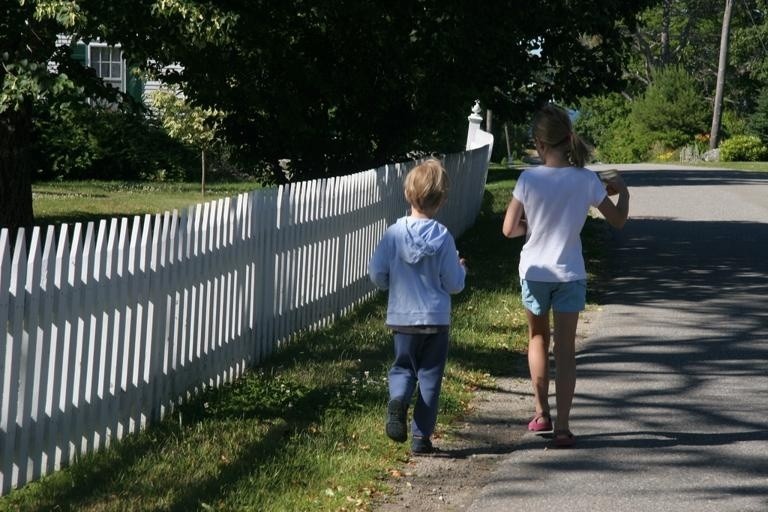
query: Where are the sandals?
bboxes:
[528,411,552,432]
[554,423,576,446]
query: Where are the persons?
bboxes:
[500,101,630,449]
[365,159,469,455]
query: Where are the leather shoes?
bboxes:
[384,398,407,443]
[412,437,432,453]
